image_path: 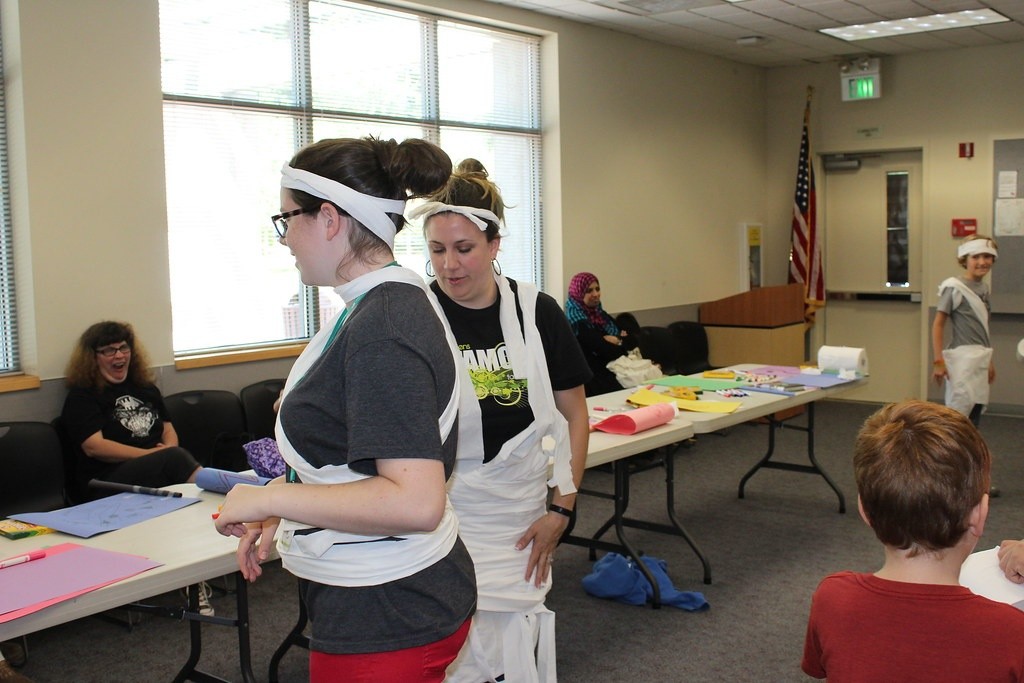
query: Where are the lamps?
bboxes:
[837,56,882,101]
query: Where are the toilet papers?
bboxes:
[817,345,869,373]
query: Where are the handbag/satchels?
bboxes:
[606,347,664,388]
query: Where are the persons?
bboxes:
[931,233,998,497]
[802,398,1023,683]
[564,272,697,451]
[62,322,215,616]
[214,139,479,683]
[407,158,590,683]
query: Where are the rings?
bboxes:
[550,559,554,562]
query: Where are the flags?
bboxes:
[789,109,826,331]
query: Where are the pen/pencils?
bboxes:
[738,374,776,383]
[716,389,748,397]
[0,550,46,570]
[757,384,784,391]
[593,406,625,412]
[646,384,655,390]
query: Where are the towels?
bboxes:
[580,552,710,613]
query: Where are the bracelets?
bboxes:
[549,503,572,517]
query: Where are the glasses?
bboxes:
[272,202,321,238]
[95,344,130,356]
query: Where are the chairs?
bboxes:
[0,415,79,521]
[163,389,249,473]
[239,378,287,442]
[616,312,710,376]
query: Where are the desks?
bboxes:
[0,482,283,683]
[238,397,694,683]
[588,362,870,585]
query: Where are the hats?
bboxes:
[957,238,998,261]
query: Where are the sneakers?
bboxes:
[186,581,215,616]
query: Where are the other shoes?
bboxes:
[988,485,999,496]
[0,652,31,683]
